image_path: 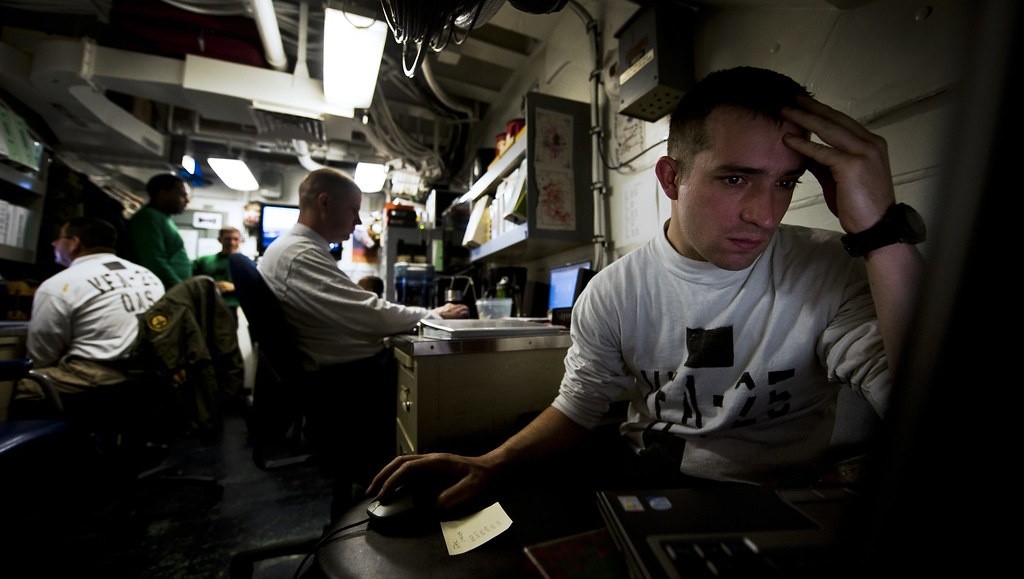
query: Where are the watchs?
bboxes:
[841,201,926,258]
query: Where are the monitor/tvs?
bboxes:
[547,260,593,312]
[257,204,342,260]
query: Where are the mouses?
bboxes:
[367,477,448,525]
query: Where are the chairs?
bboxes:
[66,275,223,499]
[0,358,116,579]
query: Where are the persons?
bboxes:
[9,173,245,430]
[364,66,926,510]
[255,168,469,451]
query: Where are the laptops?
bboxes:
[596,4,1024,579]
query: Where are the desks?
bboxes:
[316,475,628,579]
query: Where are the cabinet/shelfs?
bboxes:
[441,90,593,265]
[392,348,570,459]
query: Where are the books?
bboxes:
[460,158,531,248]
